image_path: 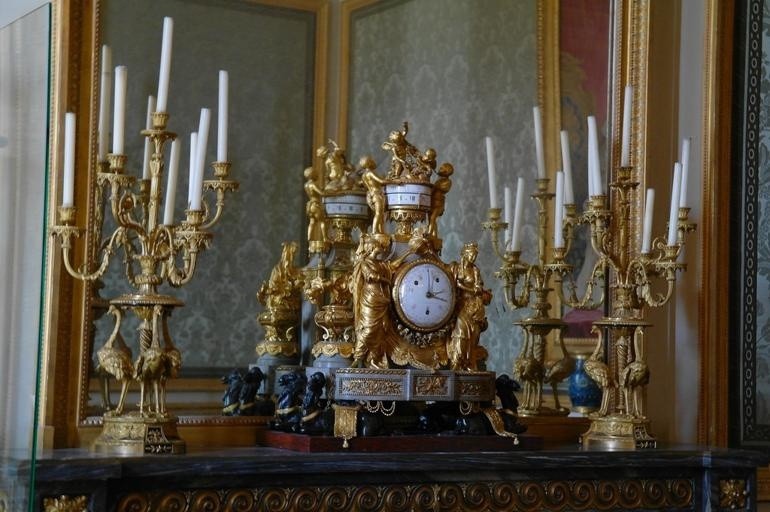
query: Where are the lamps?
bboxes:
[86,49,156,413]
[578,89,691,451]
[485,108,603,418]
[51,19,239,455]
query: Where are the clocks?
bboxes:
[221,139,370,417]
[268,122,541,454]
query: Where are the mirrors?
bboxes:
[15,0,769,512]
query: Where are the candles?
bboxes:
[62,16,231,228]
[485,107,547,252]
[99,45,181,226]
[554,88,690,255]
